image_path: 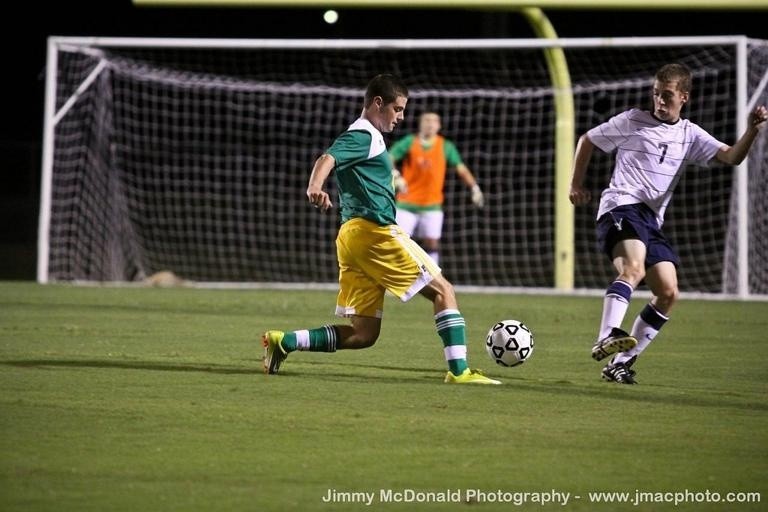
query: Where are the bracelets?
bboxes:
[470,185,481,192]
[393,170,400,176]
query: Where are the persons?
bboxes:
[384,111,485,296]
[263,74,502,385]
[570,62,767,384]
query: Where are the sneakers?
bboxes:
[444,368,503,385]
[601,358,637,384]
[591,328,637,361]
[261,331,289,375]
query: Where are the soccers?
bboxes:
[485,319,533,366]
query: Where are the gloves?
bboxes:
[470,185,483,207]
[392,168,407,192]
